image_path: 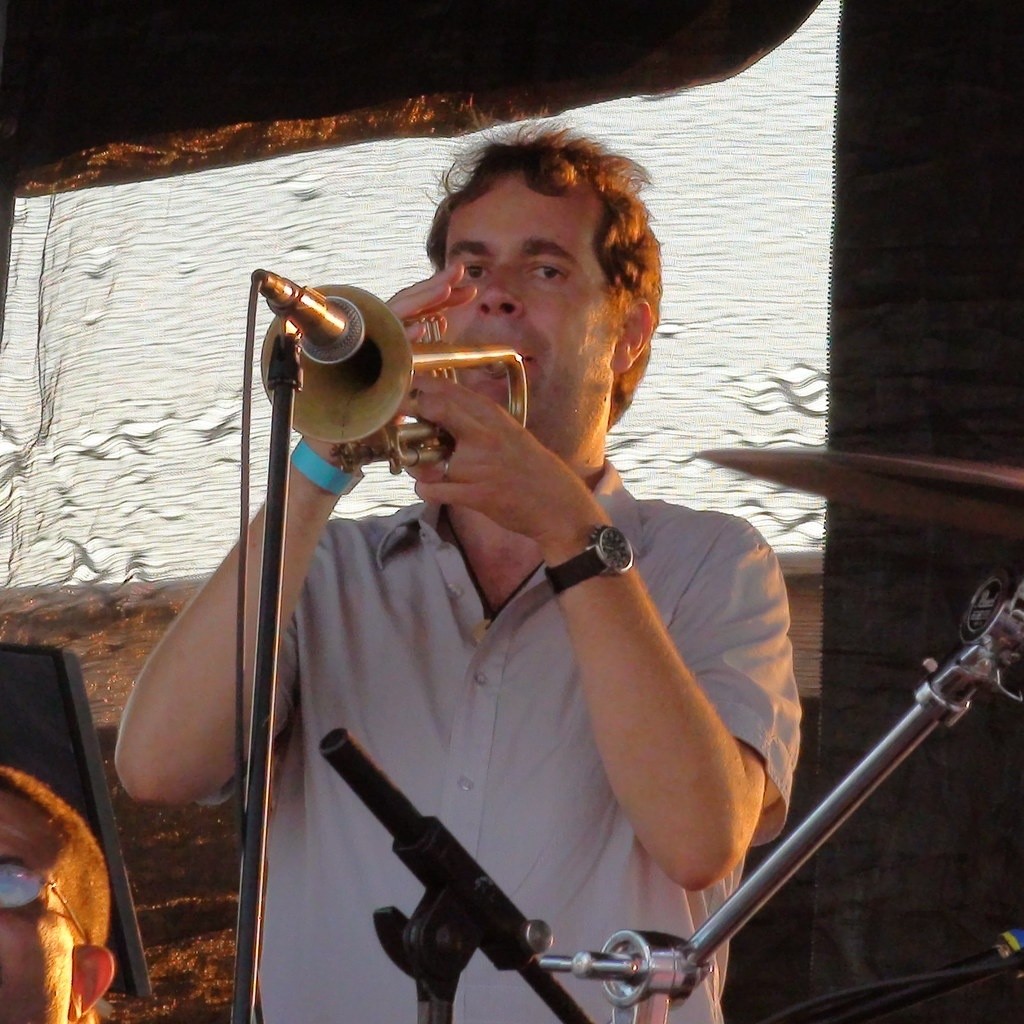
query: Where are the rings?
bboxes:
[442,463,449,484]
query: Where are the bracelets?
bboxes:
[289,438,364,496]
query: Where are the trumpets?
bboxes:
[248,273,539,476]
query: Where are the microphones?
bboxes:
[252,269,365,363]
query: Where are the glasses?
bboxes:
[0,863,89,945]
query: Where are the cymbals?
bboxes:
[686,436,1024,551]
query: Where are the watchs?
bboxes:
[545,525,634,591]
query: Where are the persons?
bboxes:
[0,765,116,1024]
[113,126,801,1023]
